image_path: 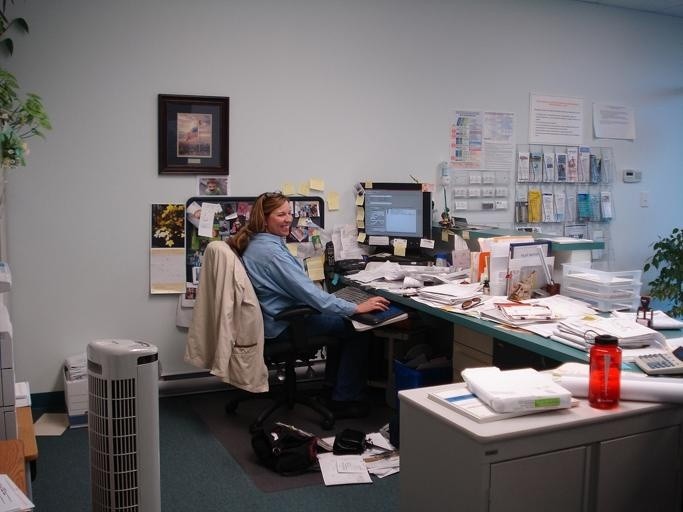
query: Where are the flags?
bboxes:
[185,125,198,143]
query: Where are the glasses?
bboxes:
[461,298,484,310]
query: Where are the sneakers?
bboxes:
[322,395,379,418]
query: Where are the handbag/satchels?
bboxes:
[332,429,368,455]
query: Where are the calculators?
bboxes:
[634,346,683,374]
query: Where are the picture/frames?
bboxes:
[157,94,229,175]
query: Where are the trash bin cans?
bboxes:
[389,358,453,451]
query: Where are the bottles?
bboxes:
[584,329,622,410]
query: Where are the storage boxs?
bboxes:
[60,361,88,428]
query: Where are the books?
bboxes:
[347,236,682,349]
[63,360,87,380]
[15,381,32,408]
[559,362,683,405]
[428,366,580,423]
[316,435,336,452]
[0,473,36,512]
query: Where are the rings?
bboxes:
[376,301,379,306]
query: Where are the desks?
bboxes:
[13,379,38,482]
[0,440,26,494]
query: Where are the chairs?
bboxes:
[227,305,340,433]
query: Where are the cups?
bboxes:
[547,283,560,296]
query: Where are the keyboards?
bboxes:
[331,286,376,305]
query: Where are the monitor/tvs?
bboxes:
[357,181,432,261]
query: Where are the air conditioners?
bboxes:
[88,338,161,512]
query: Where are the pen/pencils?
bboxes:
[403,293,419,297]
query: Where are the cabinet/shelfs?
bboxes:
[333,224,683,512]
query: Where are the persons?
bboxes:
[226,191,391,419]
[204,177,223,195]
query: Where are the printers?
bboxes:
[0,261,19,440]
